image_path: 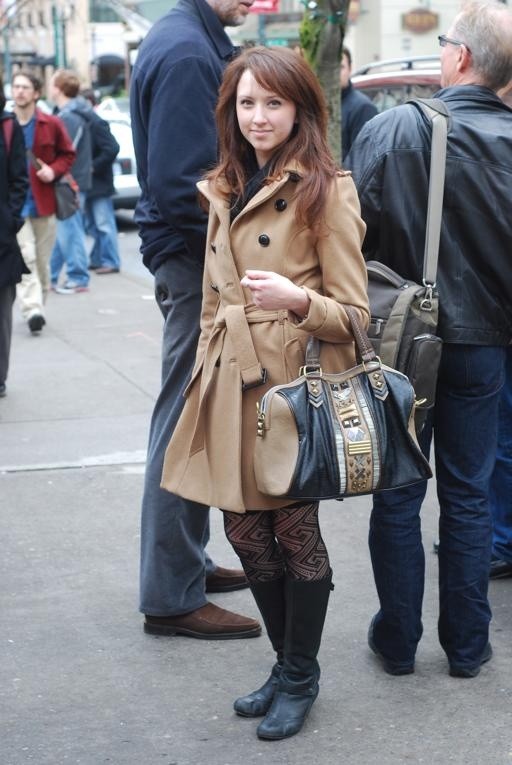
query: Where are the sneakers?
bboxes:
[488,559,512,580]
[28,314,45,336]
[450,641,492,678]
[367,614,414,676]
[45,264,118,295]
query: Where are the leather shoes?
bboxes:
[205,567,250,593]
[144,602,262,640]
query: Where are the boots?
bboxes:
[234,568,286,717]
[257,566,334,740]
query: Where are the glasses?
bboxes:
[438,35,472,55]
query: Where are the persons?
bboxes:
[10,72,75,336]
[47,67,95,298]
[129,0,269,645]
[68,87,124,278]
[341,0,511,688]
[487,344,510,579]
[156,44,373,743]
[336,45,381,163]
[0,75,33,401]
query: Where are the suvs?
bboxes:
[348,55,448,112]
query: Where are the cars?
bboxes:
[96,97,135,118]
[3,97,51,117]
[88,114,144,212]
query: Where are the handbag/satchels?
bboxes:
[253,303,433,501]
[355,260,444,439]
[52,183,79,221]
